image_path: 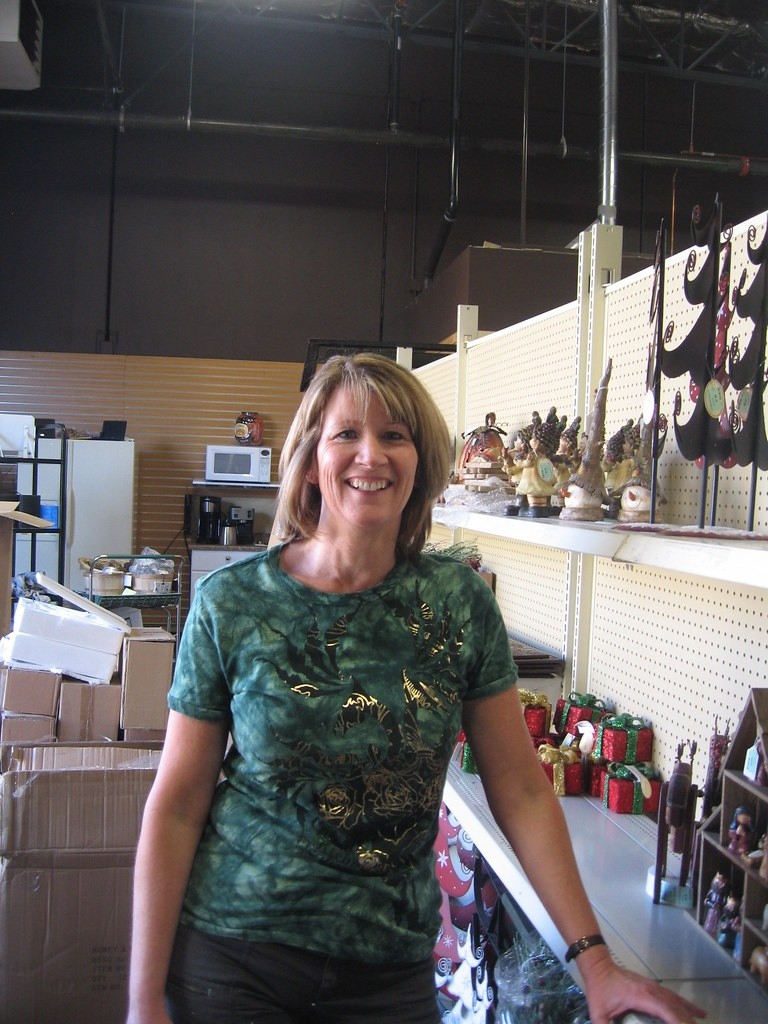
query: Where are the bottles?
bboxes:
[124,573,132,590]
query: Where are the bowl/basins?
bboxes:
[83,573,123,596]
[132,574,174,593]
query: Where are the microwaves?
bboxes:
[205,445,272,483]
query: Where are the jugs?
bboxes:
[220,525,237,545]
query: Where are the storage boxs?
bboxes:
[119,628,176,730]
[517,688,552,737]
[585,760,607,798]
[14,572,132,655]
[592,711,653,763]
[599,763,660,813]
[56,678,122,743]
[123,729,165,742]
[1,715,56,743]
[2,666,63,717]
[0,501,55,640]
[552,691,612,738]
[10,631,118,681]
[537,739,583,796]
[0,744,163,1024]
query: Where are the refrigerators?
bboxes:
[14,438,135,601]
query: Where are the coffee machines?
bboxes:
[228,507,255,546]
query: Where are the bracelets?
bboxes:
[565,933,605,963]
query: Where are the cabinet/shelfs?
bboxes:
[433,505,768,1024]
[0,423,67,605]
[185,534,271,608]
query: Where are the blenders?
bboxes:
[185,494,220,544]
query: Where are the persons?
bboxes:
[126,353,706,1023]
[497,405,670,523]
[703,738,768,962]
